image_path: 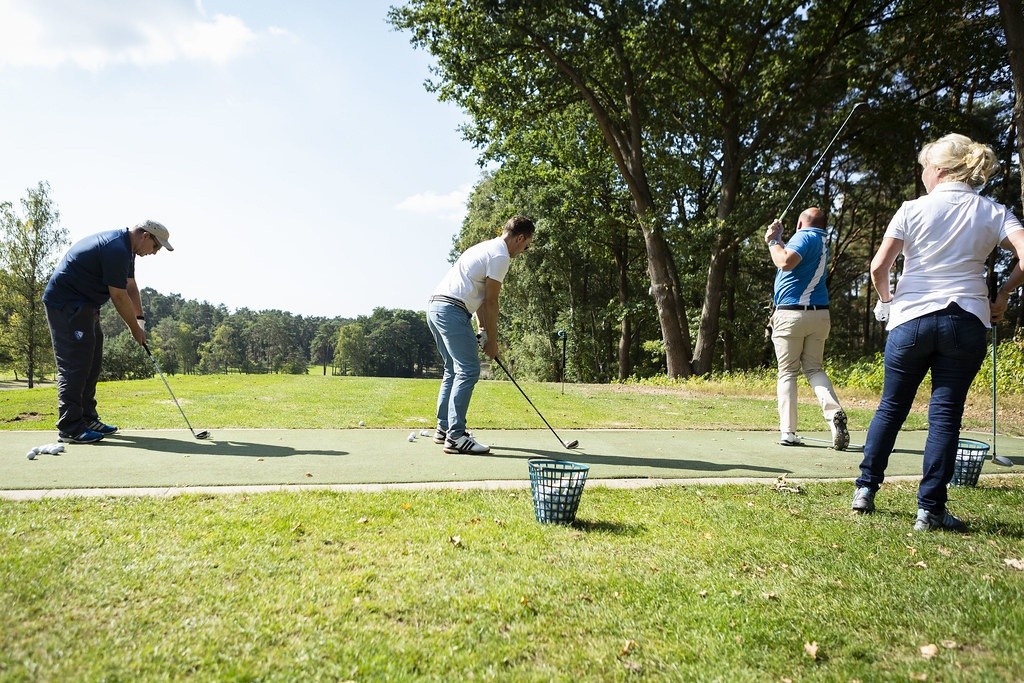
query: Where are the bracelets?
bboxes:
[997,287,1010,298]
[137,316,144,320]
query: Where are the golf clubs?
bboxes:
[478,332,579,450]
[140,340,211,440]
[780,101,871,220]
[989,323,1014,467]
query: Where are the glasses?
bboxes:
[151,237,160,251]
[524,243,529,250]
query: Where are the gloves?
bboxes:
[768,219,784,241]
[874,296,893,322]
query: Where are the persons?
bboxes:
[42,220,174,442]
[426,215,534,454]
[765,208,849,450]
[853,134,1024,530]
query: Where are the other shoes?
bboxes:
[781,433,801,446]
[833,410,851,451]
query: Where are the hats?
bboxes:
[137,220,174,251]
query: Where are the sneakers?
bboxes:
[852,486,875,511]
[443,435,490,454]
[85,417,118,435]
[434,427,447,443]
[57,431,104,444]
[915,508,969,533]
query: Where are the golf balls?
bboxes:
[359,421,364,426]
[407,429,429,443]
[945,448,987,488]
[529,476,584,524]
[28,442,67,460]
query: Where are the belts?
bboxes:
[779,305,831,311]
[431,296,467,310]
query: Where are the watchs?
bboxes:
[769,240,779,246]
